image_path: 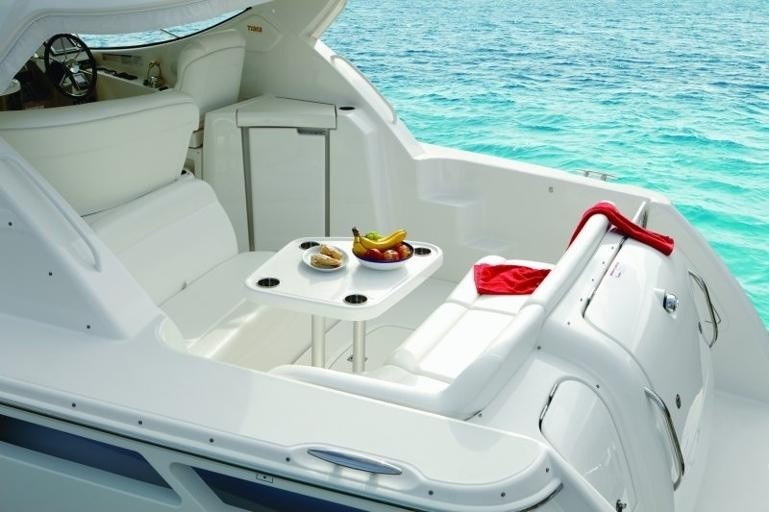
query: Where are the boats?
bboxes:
[0,0,768,511]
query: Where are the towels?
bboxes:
[472,202,676,294]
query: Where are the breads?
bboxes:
[311,244,342,268]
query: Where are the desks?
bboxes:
[243,235,444,372]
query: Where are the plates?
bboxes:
[301,244,348,272]
[351,238,415,268]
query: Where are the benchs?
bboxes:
[87,171,342,367]
[373,199,614,421]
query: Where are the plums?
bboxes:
[364,246,410,263]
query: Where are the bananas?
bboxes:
[352,226,407,257]
